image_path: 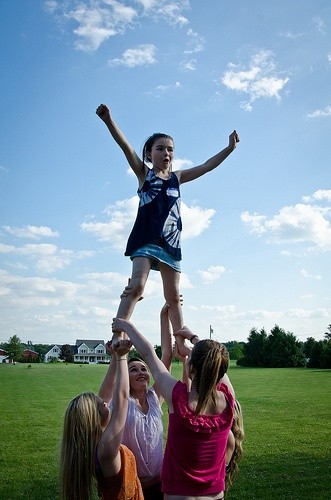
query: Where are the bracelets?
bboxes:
[116,358,127,361]
[190,334,199,344]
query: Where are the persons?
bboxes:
[97,278,184,500]
[175,340,246,499]
[112,318,236,500]
[60,339,144,499]
[173,337,245,500]
[95,102,241,357]
